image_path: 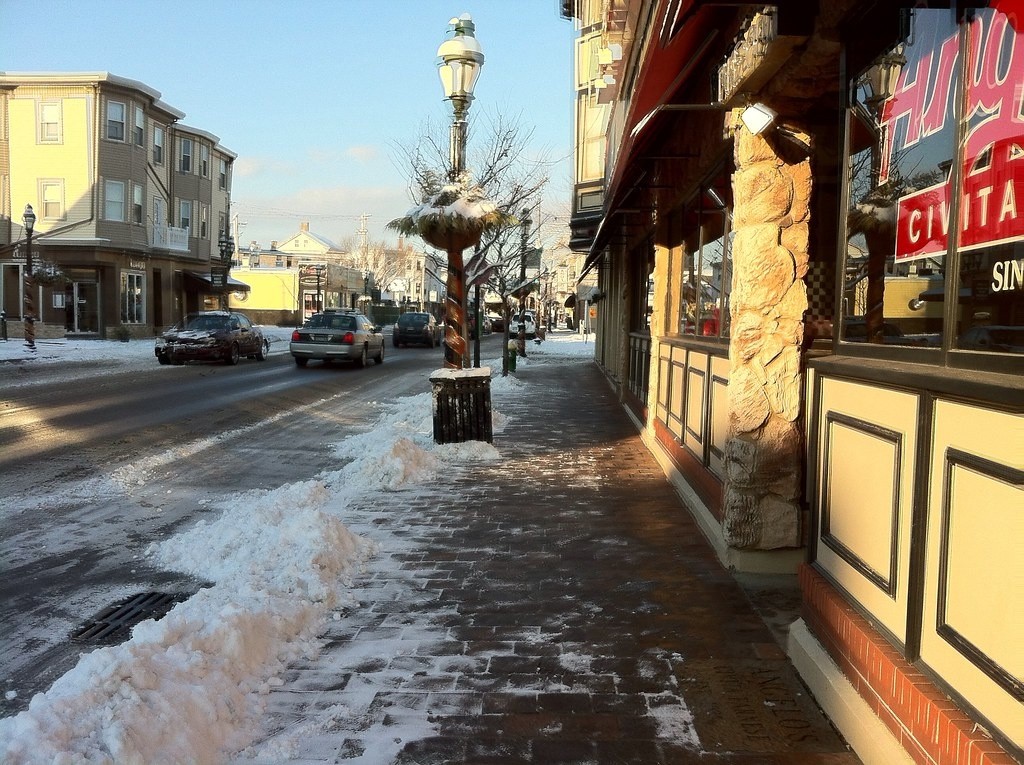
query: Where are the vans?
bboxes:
[520,309,537,323]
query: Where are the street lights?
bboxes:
[21,203,36,346]
[518,207,534,357]
[313,263,323,313]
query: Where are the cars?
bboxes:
[482,316,493,335]
[290,308,384,368]
[392,312,443,349]
[489,317,508,333]
[155,310,269,366]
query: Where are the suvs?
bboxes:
[435,13,485,369]
[508,311,537,340]
